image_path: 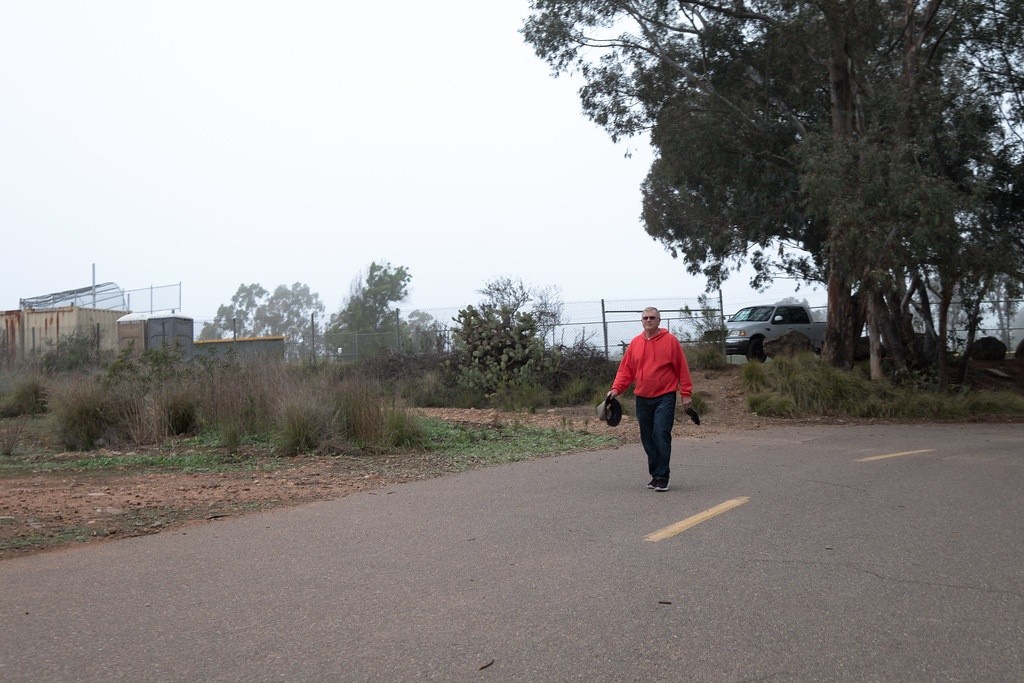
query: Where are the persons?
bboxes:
[607,306,693,492]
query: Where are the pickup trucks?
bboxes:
[720,305,828,365]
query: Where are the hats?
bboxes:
[597,394,622,427]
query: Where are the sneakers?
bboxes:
[647,476,671,491]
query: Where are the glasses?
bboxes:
[642,317,656,321]
